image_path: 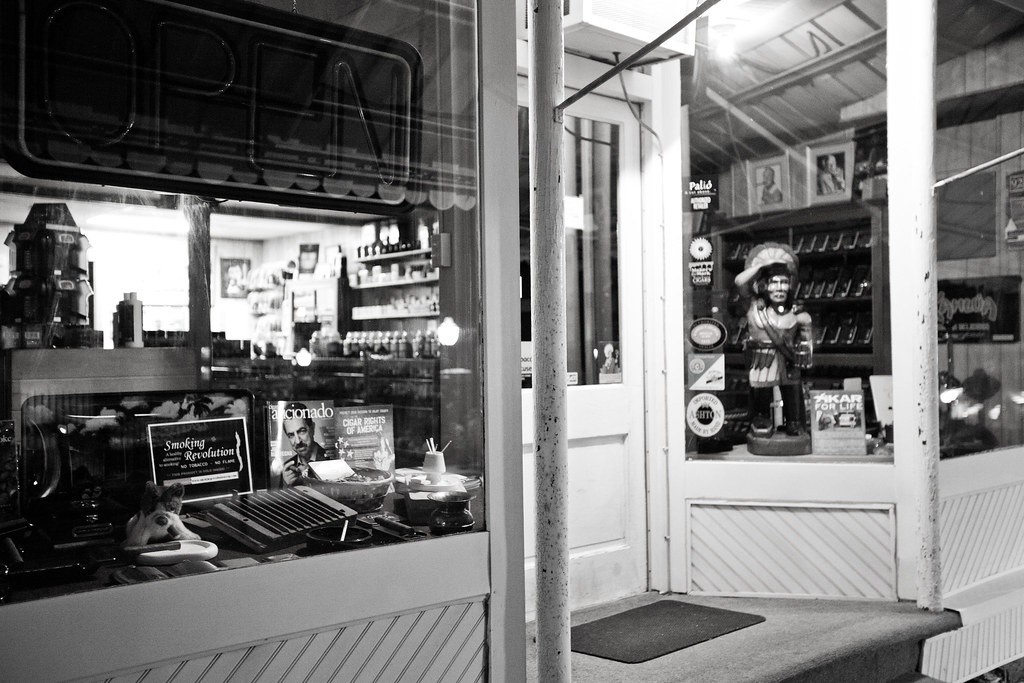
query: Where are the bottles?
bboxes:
[121,292,142,348]
[309,330,440,358]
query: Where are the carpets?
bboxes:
[532,600,767,664]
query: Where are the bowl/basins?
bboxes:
[306,527,373,553]
[301,465,394,509]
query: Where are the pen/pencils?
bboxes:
[426,437,452,453]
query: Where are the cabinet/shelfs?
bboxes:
[708,206,883,388]
[351,247,438,319]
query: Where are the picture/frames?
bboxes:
[810,142,855,204]
[749,155,789,214]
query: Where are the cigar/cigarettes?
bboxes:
[288,466,300,473]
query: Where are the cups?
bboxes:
[427,491,477,532]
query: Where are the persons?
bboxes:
[279,403,330,489]
[817,154,844,195]
[760,164,782,206]
[733,260,816,432]
